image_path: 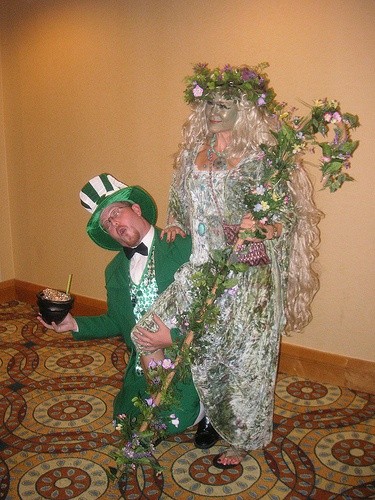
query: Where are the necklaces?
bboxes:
[207,136,232,160]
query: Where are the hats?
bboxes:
[79,173,158,252]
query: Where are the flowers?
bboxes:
[106,62,360,490]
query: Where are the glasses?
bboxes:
[102,206,131,231]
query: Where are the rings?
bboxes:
[146,343,149,348]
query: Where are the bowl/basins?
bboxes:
[36,290,75,325]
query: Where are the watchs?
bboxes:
[272,224,277,238]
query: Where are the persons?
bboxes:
[130,59,324,469]
[37,172,220,450]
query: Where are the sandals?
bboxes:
[213,445,252,470]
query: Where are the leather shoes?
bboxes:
[194,416,219,449]
